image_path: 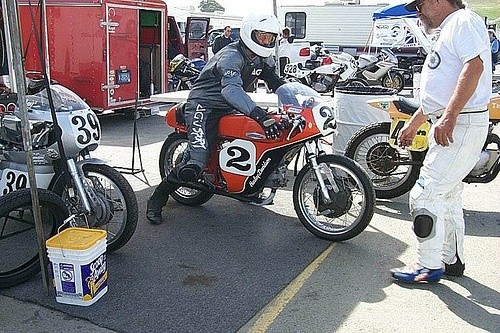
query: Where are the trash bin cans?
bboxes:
[332,87,398,182]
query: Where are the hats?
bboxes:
[404,0,422,12]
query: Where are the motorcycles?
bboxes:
[344,79,499,202]
[159,82,376,242]
[282,48,405,92]
[0,72,141,252]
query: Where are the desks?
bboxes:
[150,90,330,176]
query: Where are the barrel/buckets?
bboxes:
[46,212,109,305]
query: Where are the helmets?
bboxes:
[240,14,284,57]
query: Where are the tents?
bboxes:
[364,3,419,55]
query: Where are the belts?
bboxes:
[437,109,489,119]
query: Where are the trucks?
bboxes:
[11,0,210,119]
[277,2,426,64]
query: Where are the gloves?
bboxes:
[257,111,284,140]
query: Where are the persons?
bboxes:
[215,26,290,95]
[390,0,500,284]
[146,14,306,224]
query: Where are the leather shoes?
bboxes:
[252,192,274,204]
[146,198,164,225]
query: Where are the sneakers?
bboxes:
[443,259,465,277]
[389,263,444,284]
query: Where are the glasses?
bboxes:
[413,0,425,13]
[254,30,276,43]
